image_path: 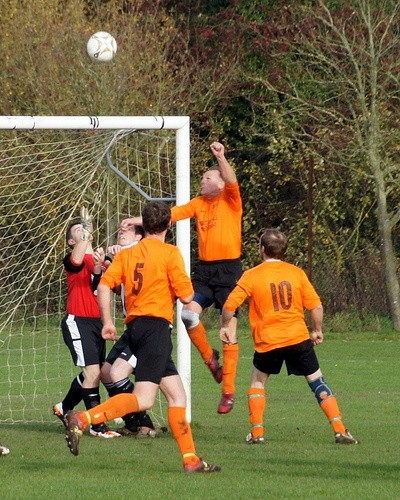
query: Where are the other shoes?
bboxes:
[217,392,234,414]
[204,348,223,384]
[184,457,221,473]
[108,427,139,436]
[244,432,265,444]
[335,429,361,444]
[52,402,73,428]
[136,426,154,439]
[64,410,87,456]
[89,423,121,439]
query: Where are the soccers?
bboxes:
[87,31,117,62]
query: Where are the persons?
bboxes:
[219,229,360,445]
[65,201,221,473]
[99,225,156,439]
[52,206,123,439]
[120,141,244,414]
